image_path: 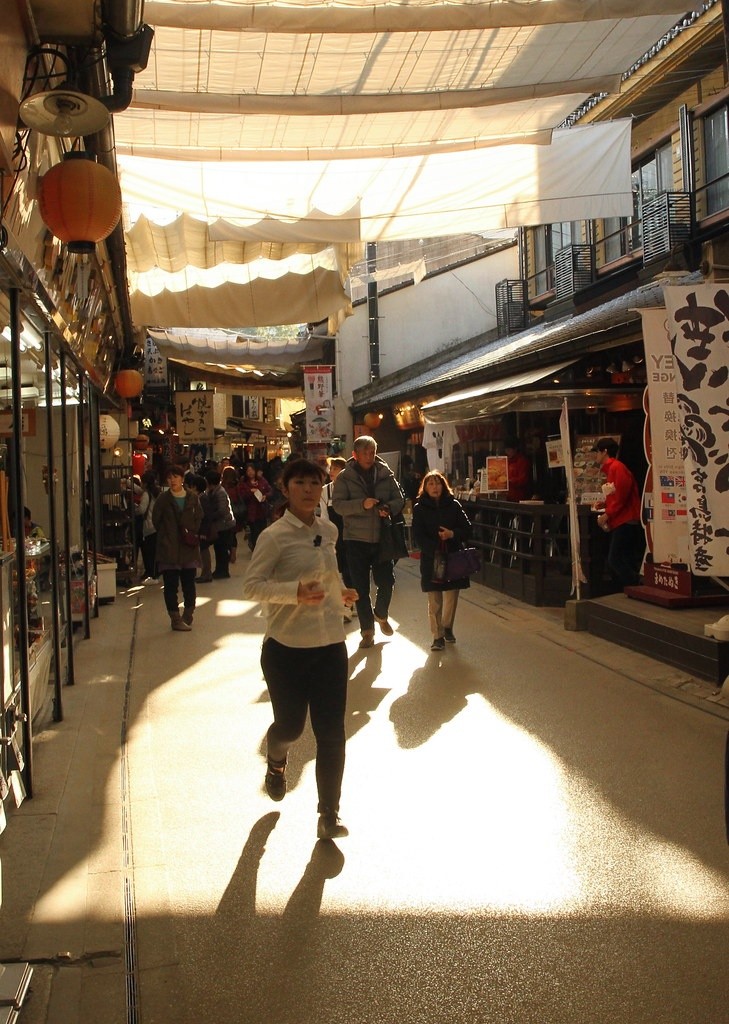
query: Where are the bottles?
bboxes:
[597,515,609,532]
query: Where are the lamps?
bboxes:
[18,48,110,139]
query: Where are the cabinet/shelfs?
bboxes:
[84,464,136,584]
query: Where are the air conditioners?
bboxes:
[649,223,689,257]
[562,269,589,295]
[503,301,523,327]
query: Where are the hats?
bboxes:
[590,438,618,452]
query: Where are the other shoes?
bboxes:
[373,614,393,636]
[359,635,374,647]
[139,544,255,594]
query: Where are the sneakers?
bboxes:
[265,762,287,802]
[442,628,456,643]
[352,602,358,616]
[342,607,352,622]
[317,814,350,839]
[431,636,445,650]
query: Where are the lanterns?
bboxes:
[39,150,124,255]
[99,410,121,454]
[365,410,381,432]
[135,433,150,450]
[117,369,144,419]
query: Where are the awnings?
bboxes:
[226,417,288,444]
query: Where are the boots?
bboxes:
[181,607,193,625]
[169,611,192,631]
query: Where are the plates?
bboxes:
[574,444,594,478]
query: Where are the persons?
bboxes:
[502,437,530,500]
[316,454,360,620]
[590,437,643,589]
[241,458,359,839]
[410,469,475,650]
[332,436,405,649]
[132,474,162,584]
[23,505,49,586]
[151,464,205,632]
[182,451,300,583]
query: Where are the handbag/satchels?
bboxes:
[431,539,449,584]
[177,525,200,545]
[452,540,480,576]
[380,513,409,561]
